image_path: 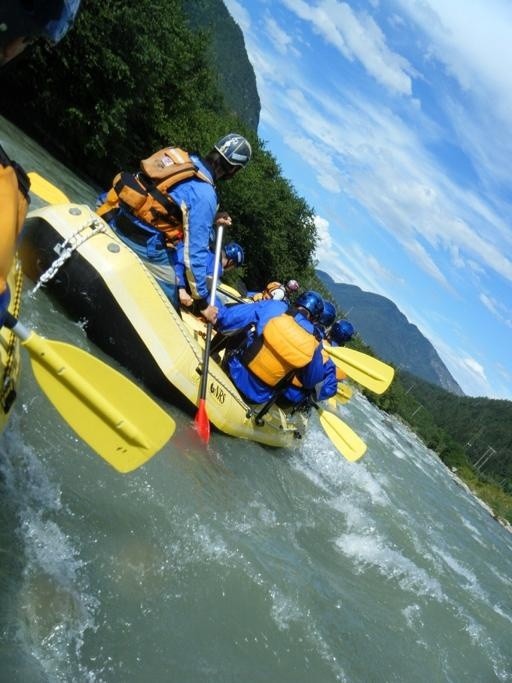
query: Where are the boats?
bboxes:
[0,251,24,438]
[18,200,312,451]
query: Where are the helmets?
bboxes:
[329,319,356,344]
[294,288,325,322]
[317,301,338,329]
[0,0,81,48]
[214,133,252,170]
[222,241,245,267]
[287,279,300,291]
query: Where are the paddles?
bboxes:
[193,224,223,443]
[25,171,69,206]
[0,306,176,473]
[217,287,394,395]
[334,367,346,379]
[327,396,338,409]
[336,382,354,404]
[313,403,367,462]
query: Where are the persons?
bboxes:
[97,127,257,329]
[275,299,340,410]
[206,235,249,282]
[326,318,354,348]
[3,2,91,322]
[253,279,300,302]
[215,290,326,399]
